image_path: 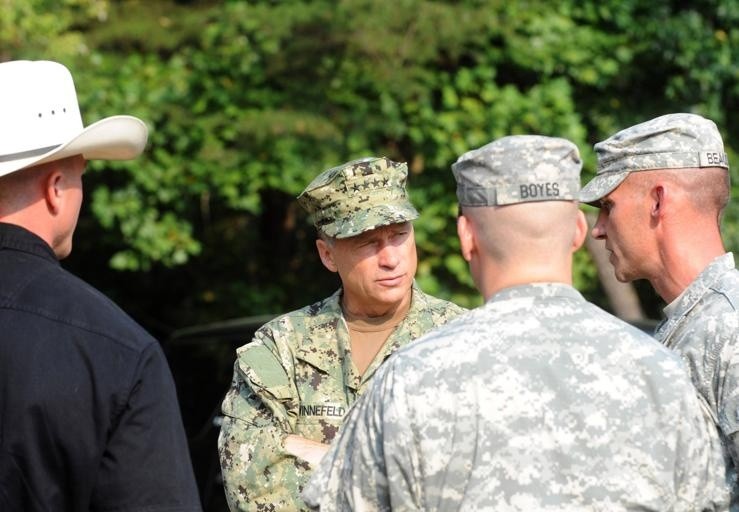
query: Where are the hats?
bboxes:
[579,112,727,207]
[294,158,420,240]
[0,61,148,182]
[451,135,585,207]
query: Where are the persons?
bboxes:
[297,134,734,512]
[578,111,739,512]
[215,154,472,512]
[0,58,204,511]
